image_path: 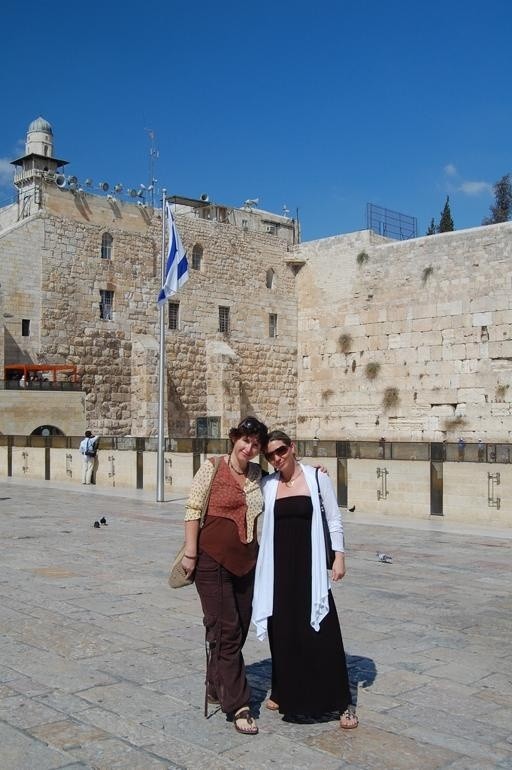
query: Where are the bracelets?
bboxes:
[184,555,197,560]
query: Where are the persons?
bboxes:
[78,431,100,486]
[250,431,359,729]
[19,375,28,388]
[32,375,38,382]
[44,378,48,382]
[181,417,268,734]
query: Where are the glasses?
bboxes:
[239,420,268,440]
[265,445,291,462]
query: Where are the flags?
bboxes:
[158,199,189,310]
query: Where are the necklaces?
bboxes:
[229,459,248,475]
[281,471,301,488]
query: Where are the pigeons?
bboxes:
[376,551,392,563]
[100,517,108,525]
[94,522,100,528]
[349,505,355,512]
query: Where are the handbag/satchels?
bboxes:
[315,466,335,570]
[167,540,195,590]
[85,450,97,457]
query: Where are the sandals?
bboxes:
[266,698,279,710]
[339,707,359,729]
[231,707,259,735]
